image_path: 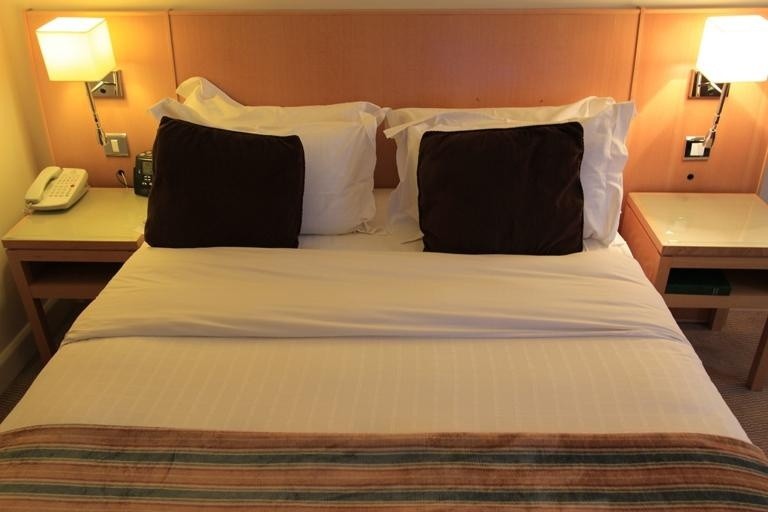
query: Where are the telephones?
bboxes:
[24,166,90,210]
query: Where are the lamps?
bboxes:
[33,16,122,147]
[680,11,768,157]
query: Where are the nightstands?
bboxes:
[0,188,154,364]
[622,191,768,393]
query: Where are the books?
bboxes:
[665,268,733,297]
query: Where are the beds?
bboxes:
[0,184,768,511]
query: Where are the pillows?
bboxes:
[411,120,586,258]
[172,80,378,242]
[387,97,625,240]
[152,119,299,247]
